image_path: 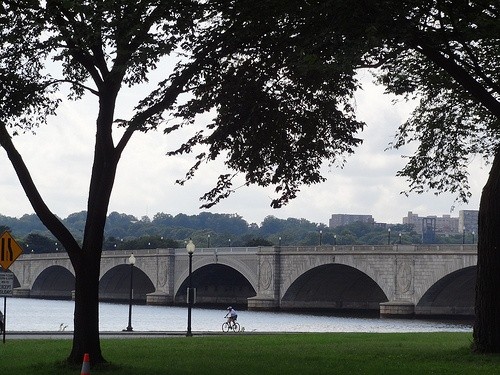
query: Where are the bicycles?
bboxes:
[221,316,241,333]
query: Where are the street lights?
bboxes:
[55,248,59,253]
[26,243,29,254]
[54,242,57,253]
[160,234,164,248]
[228,238,231,247]
[114,244,117,250]
[462,225,465,244]
[333,234,336,245]
[120,237,123,250]
[184,239,187,248]
[471,231,475,244]
[185,237,195,337]
[148,241,151,249]
[399,233,402,244]
[127,251,136,332]
[278,236,282,246]
[387,227,391,245]
[207,234,210,248]
[319,229,323,246]
[31,249,34,254]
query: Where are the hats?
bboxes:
[227,307,232,309]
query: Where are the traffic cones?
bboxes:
[80,353,91,375]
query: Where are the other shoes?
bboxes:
[235,325,237,329]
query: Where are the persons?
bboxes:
[224,307,238,328]
